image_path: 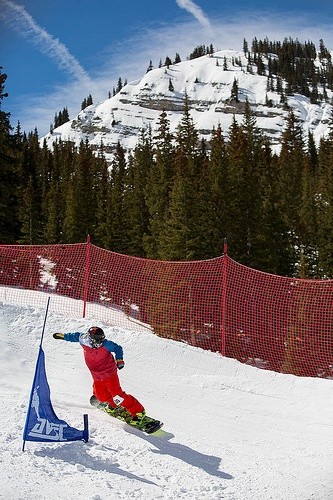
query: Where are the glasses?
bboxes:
[94,337,106,343]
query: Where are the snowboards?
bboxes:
[90,395,164,435]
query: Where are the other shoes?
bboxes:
[106,403,122,413]
[128,410,146,425]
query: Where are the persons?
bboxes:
[53,326,146,426]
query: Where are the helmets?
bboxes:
[88,327,106,343]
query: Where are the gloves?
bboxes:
[116,359,125,370]
[52,332,64,340]
